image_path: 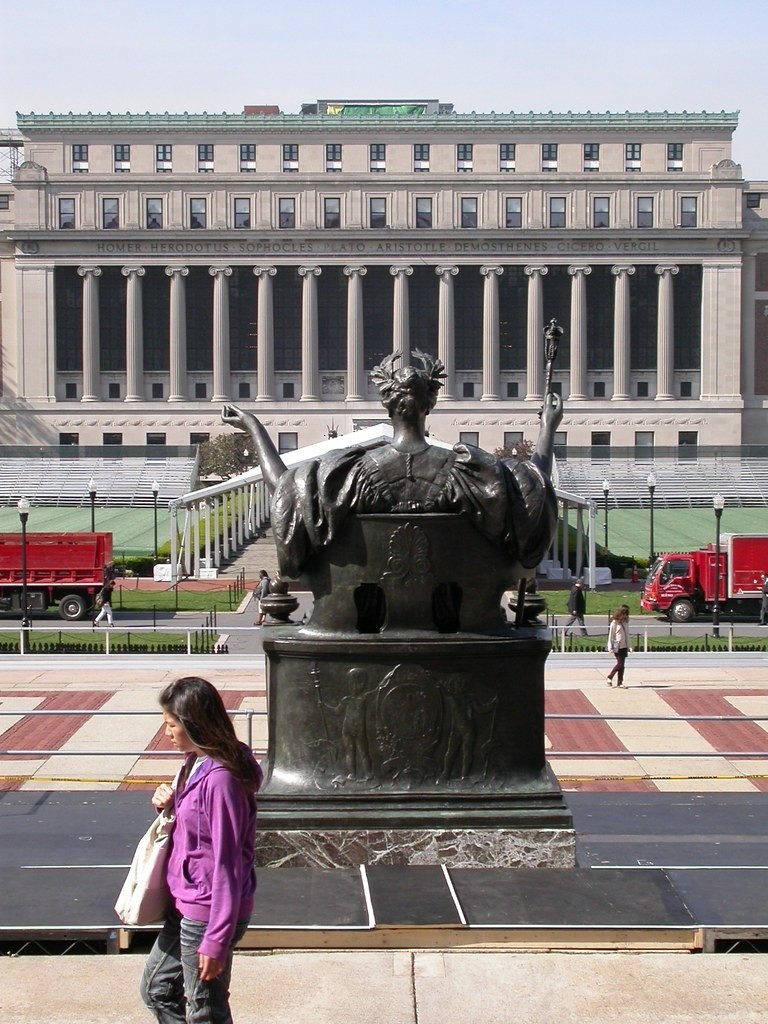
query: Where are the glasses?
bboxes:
[625,612,629,616]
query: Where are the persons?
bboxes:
[252,569,271,625]
[758,572,768,625]
[564,579,589,638]
[139,677,265,1024]
[605,605,632,686]
[221,348,562,633]
[92,580,116,629]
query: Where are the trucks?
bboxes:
[0,531,115,621]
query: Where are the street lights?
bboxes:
[601,479,611,556]
[152,480,160,565]
[646,472,658,565]
[17,494,30,653]
[708,492,725,638]
[87,478,97,533]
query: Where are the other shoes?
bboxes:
[607,677,612,686]
[253,621,262,626]
[617,684,628,690]
[757,622,767,625]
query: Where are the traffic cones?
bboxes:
[629,565,641,584]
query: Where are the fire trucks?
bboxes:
[636,531,768,623]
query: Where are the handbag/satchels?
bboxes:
[95,588,105,606]
[113,767,177,925]
[253,579,262,601]
